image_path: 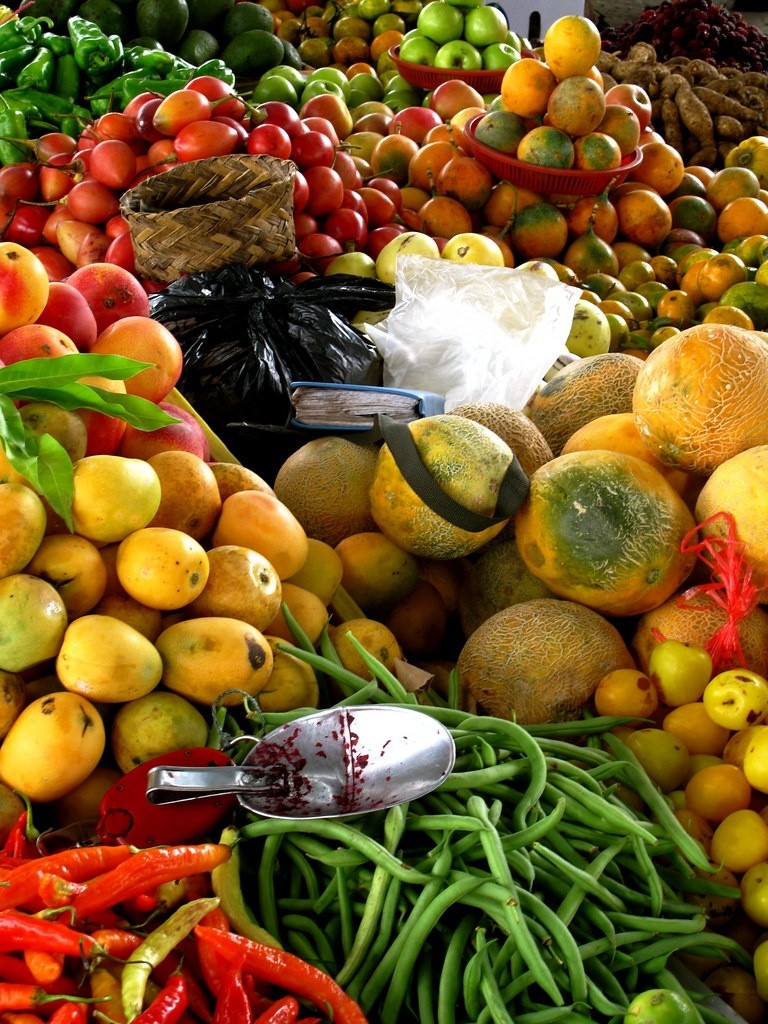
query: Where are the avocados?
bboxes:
[0,0,306,84]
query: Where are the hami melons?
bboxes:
[277,323,768,727]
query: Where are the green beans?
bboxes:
[202,600,756,1024]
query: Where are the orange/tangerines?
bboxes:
[344,79,768,358]
[238,0,427,79]
[500,15,604,135]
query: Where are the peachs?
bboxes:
[0,238,209,465]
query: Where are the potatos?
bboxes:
[592,43,768,170]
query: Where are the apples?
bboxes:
[604,83,652,134]
[592,640,768,1024]
[245,66,423,119]
[391,0,524,141]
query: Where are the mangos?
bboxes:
[0,404,458,846]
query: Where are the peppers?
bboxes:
[0,790,368,1024]
[0,5,236,170]
